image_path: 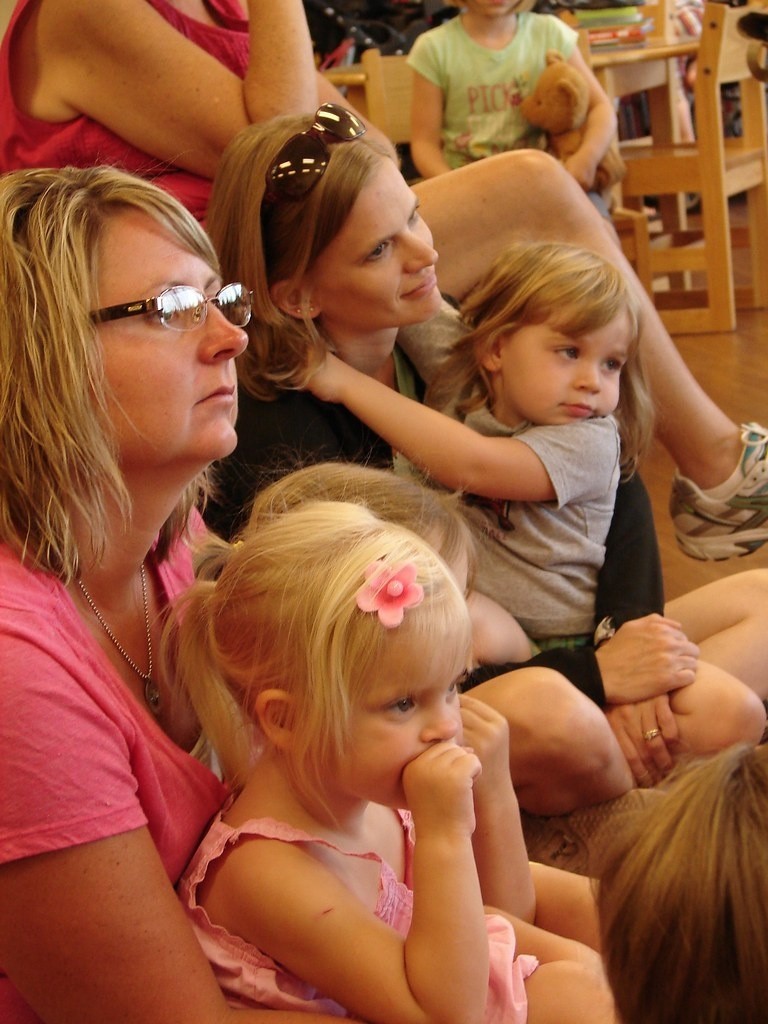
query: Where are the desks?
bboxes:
[320,32,701,294]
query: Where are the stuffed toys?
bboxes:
[519,48,629,193]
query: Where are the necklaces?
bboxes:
[63,553,172,722]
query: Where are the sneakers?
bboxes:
[669,421,768,562]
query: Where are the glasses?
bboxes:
[258,102,367,241]
[88,282,255,332]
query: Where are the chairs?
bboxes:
[606,206,653,310]
[359,45,423,187]
[617,0,767,337]
[556,0,679,211]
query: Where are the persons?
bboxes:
[403,0,619,240]
[0,0,768,564]
[290,236,653,690]
[137,499,546,1024]
[202,109,768,809]
[0,161,629,1024]
